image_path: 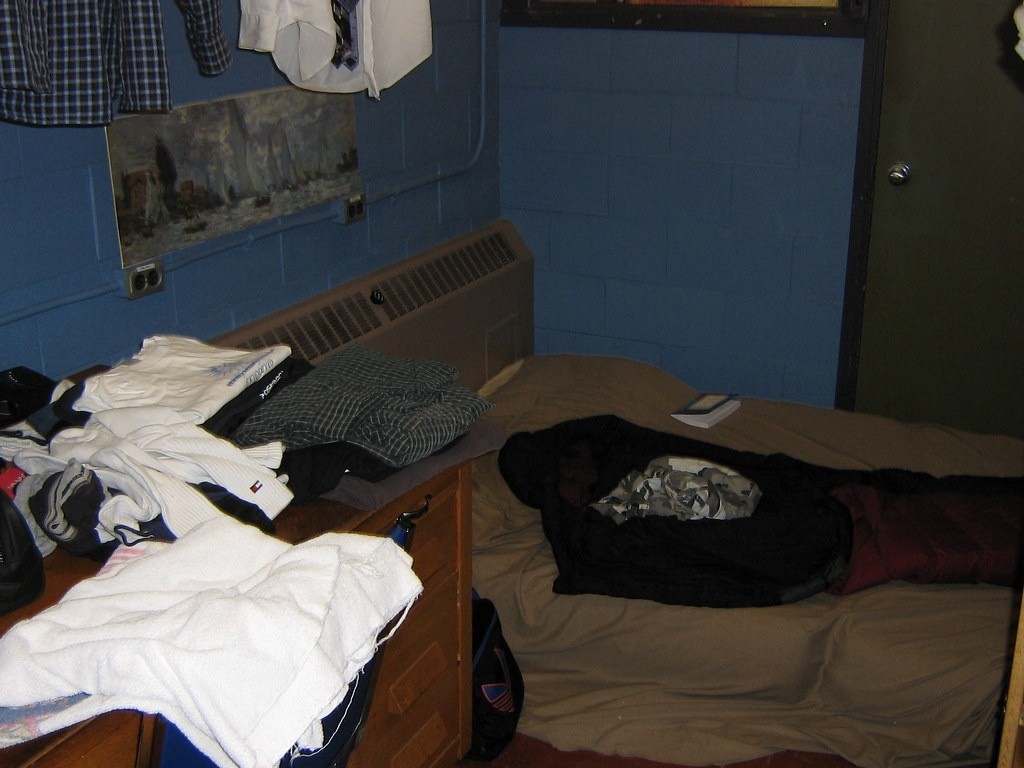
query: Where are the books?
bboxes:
[670,393,741,429]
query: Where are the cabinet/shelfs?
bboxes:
[0,460,473,767]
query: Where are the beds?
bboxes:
[201,217,1024,768]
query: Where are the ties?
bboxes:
[331,0,360,72]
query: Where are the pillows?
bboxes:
[470,354,700,551]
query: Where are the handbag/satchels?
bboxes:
[464,587,524,761]
